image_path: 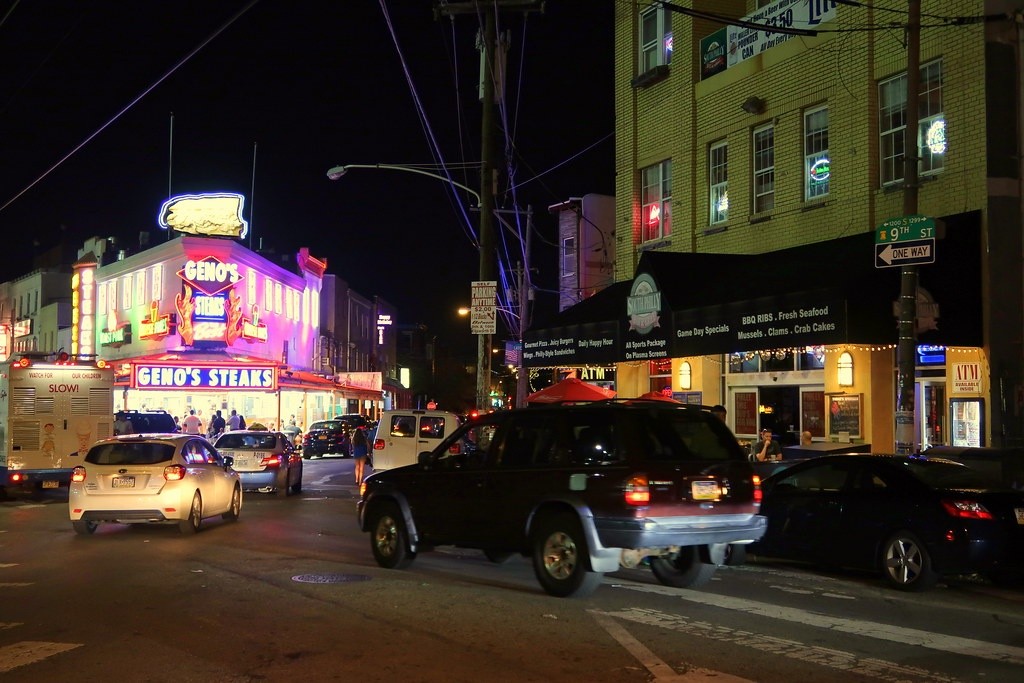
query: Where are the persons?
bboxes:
[208,410,225,438]
[227,410,246,431]
[174,410,202,435]
[351,428,371,486]
[280,414,303,437]
[755,429,782,461]
[115,411,133,435]
[269,423,275,431]
[801,431,812,445]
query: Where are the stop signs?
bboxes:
[427,402,436,411]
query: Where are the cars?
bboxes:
[280,426,302,449]
[211,429,303,497]
[744,444,1024,592]
[303,420,352,460]
[68,432,241,535]
[334,413,378,454]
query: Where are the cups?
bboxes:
[770,455,775,462]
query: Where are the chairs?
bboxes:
[501,426,615,465]
[106,449,124,463]
[154,450,169,464]
[225,438,242,449]
[260,438,276,448]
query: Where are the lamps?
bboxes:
[838,352,853,386]
[321,358,331,365]
[741,95,760,113]
[679,361,691,389]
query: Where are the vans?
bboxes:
[372,409,464,473]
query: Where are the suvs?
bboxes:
[113,407,182,436]
[355,397,769,597]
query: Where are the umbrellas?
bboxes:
[624,391,680,406]
[522,377,617,405]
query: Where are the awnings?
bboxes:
[522,209,984,368]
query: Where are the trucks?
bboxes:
[0,349,115,502]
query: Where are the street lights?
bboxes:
[458,306,522,369]
[327,163,488,410]
